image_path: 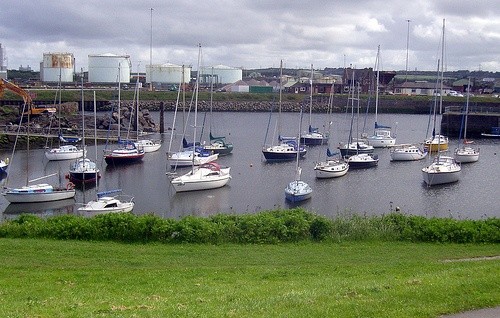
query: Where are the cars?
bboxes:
[120,85,129,90]
[447,91,474,97]
[216,88,226,92]
[169,85,177,91]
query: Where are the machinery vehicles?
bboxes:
[0,78,56,118]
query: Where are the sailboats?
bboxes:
[284,105,312,204]
[44,67,88,161]
[423,59,449,153]
[165,43,233,193]
[0,68,135,219]
[454,76,481,164]
[421,18,461,190]
[261,44,397,179]
[103,63,162,167]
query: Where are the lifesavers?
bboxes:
[67,182,74,190]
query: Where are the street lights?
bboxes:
[404,19,413,95]
[146,8,156,91]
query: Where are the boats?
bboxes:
[391,146,428,161]
